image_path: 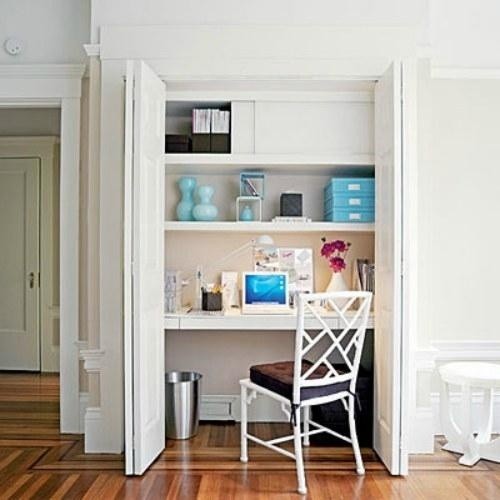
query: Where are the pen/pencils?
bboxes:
[200,282,227,293]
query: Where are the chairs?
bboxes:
[231,288,373,497]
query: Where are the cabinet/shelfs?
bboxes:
[158,88,379,334]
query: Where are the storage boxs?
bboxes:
[322,174,375,223]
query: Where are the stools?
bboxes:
[434,362,500,470]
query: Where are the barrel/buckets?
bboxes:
[352,256,374,310]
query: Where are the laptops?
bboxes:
[241,271,291,315]
